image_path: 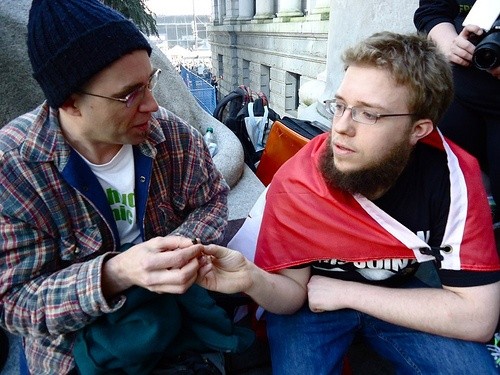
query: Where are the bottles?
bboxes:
[203,127,218,158]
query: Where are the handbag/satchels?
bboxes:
[71,282,259,375]
[244,102,274,154]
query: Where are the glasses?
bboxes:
[323,98,416,126]
[80,67,162,108]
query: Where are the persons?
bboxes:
[413,1,499,205]
[0,0,267,375]
[211,76,221,104]
[193,32,499,375]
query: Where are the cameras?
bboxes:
[468,20,500,70]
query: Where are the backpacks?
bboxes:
[212,85,267,132]
[233,97,281,171]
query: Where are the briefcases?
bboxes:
[256,115,332,187]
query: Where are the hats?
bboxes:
[26,0,153,109]
